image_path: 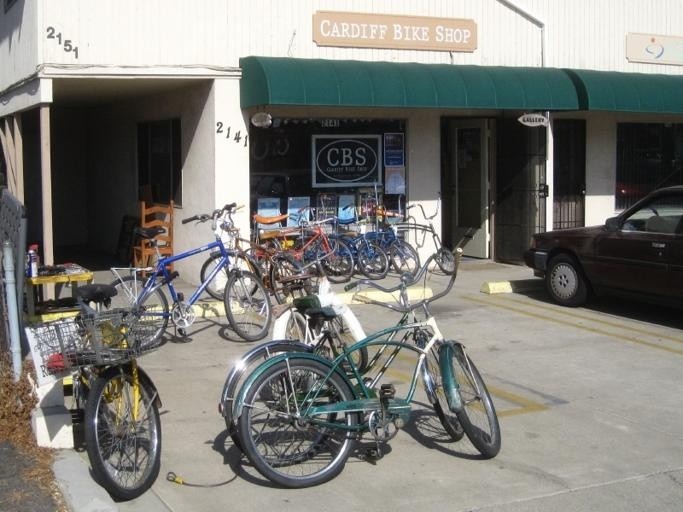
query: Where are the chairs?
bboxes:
[131,200,174,278]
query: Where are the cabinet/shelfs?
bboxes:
[25,262,96,386]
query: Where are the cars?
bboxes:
[524,185,683,307]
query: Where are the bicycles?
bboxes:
[30,284,164,501]
[221,249,501,488]
[201,193,459,300]
[272,236,368,373]
[107,204,272,347]
